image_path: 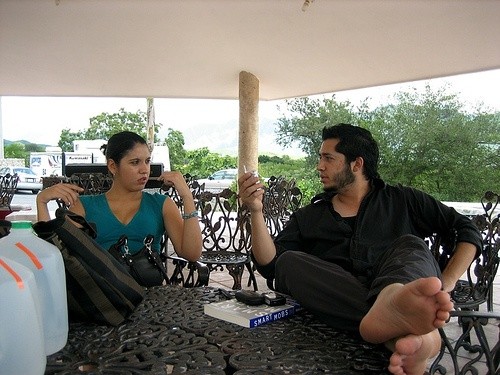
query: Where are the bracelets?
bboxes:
[182,210,198,219]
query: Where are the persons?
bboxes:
[36,131,203,375]
[238,123,484,375]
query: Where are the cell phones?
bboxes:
[235,290,286,307]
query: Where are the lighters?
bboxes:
[219,288,233,300]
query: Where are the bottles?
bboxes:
[0,220,69,355]
[0,256,47,375]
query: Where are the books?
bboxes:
[204,289,306,328]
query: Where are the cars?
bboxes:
[189,168,279,200]
[0,166,43,195]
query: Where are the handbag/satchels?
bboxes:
[33,207,145,328]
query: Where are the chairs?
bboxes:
[0,173,500,375]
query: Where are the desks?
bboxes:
[45,285,394,375]
